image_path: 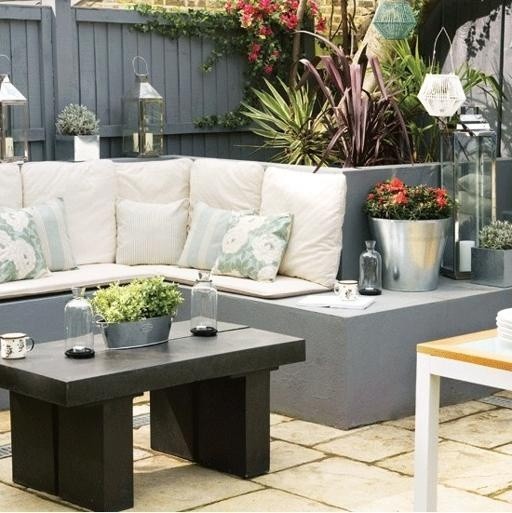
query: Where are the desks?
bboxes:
[413,327,512,513]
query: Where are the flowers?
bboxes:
[365,176,462,220]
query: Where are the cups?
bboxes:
[334,280,358,301]
[0,332,36,361]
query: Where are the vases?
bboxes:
[367,214,452,292]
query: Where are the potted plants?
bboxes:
[54,103,101,162]
[470,219,512,288]
[88,275,185,351]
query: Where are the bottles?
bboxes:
[359,240,382,294]
[190,280,218,335]
[63,287,95,357]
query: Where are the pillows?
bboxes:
[0,206,52,284]
[21,160,116,264]
[210,209,292,283]
[0,162,23,209]
[27,194,79,272]
[188,157,264,216]
[177,200,255,270]
[115,196,190,266]
[114,157,194,203]
[259,166,348,287]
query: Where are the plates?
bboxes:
[495,308,511,342]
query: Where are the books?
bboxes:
[295,292,377,311]
